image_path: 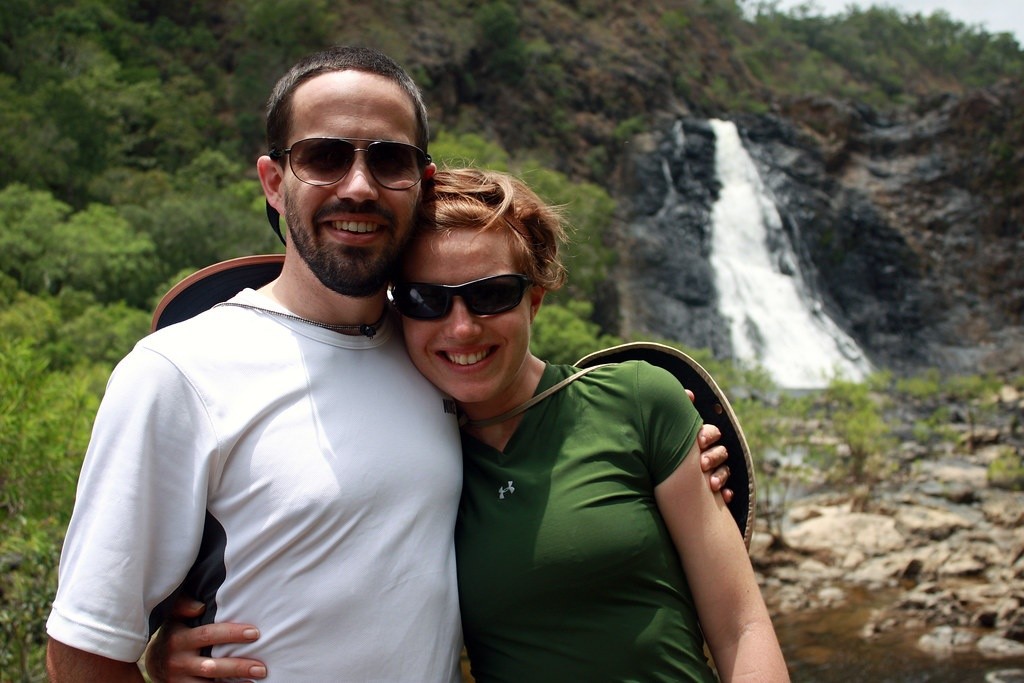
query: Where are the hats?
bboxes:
[572,341,756,550]
[150,254,290,331]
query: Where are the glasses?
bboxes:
[282,138,433,190]
[390,273,537,321]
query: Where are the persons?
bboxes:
[46,46,735,682]
[144,167,792,683]
